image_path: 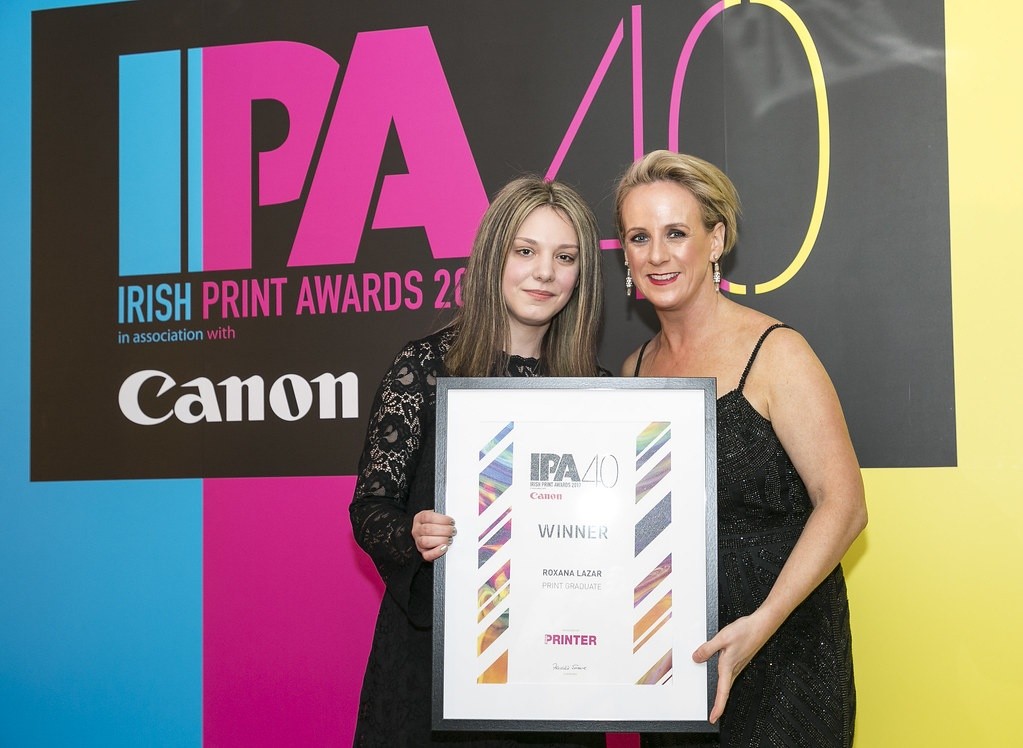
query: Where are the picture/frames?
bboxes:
[432,376,722,732]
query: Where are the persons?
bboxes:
[612,149,869,748]
[349,171,615,748]
